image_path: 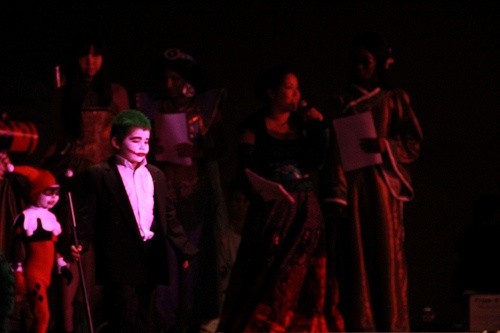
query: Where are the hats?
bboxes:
[4,164,60,196]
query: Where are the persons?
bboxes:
[0,27,423,333]
[69,109,198,333]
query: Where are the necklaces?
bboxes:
[272,115,287,128]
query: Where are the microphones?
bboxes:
[300,99,329,128]
